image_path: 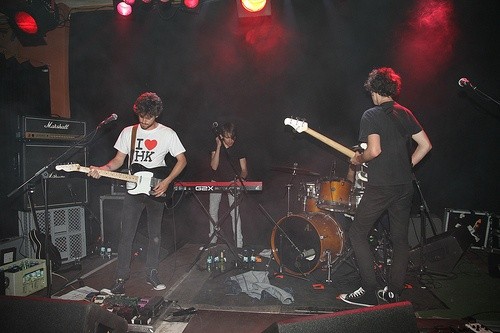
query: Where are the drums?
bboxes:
[297,193,335,220]
[317,174,355,215]
[270,208,346,279]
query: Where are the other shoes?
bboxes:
[199,243,218,251]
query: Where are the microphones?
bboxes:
[213,122,219,127]
[458,78,471,87]
[97,113,118,128]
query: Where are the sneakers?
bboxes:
[377,285,400,304]
[145,271,167,291]
[99,279,126,297]
[339,287,378,307]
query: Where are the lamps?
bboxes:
[236,0,272,19]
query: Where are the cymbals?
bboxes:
[272,165,320,177]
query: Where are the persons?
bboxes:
[199,121,247,256]
[340,67,432,306]
[88,92,187,296]
[346,162,374,196]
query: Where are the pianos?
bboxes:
[174,180,264,269]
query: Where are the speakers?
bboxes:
[91,196,124,251]
[0,137,89,211]
[406,231,470,275]
[264,301,416,333]
[0,296,128,333]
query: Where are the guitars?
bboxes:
[280,118,356,160]
[28,189,63,272]
[55,162,176,206]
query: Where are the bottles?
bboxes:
[206,249,225,273]
[243,250,250,268]
[99,247,112,261]
[7,261,45,283]
[249,249,256,268]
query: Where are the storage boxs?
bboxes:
[0,258,52,297]
